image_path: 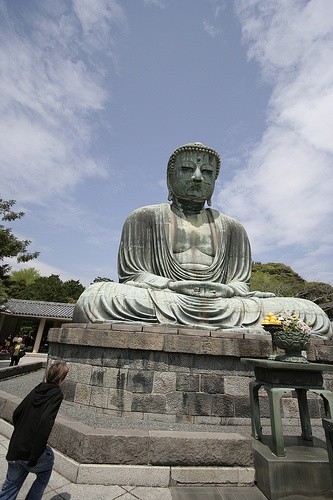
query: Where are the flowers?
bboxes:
[279,316,309,331]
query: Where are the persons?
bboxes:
[0,328,48,368]
[0,358,69,500]
[116,141,254,298]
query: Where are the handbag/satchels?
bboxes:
[19,351,26,357]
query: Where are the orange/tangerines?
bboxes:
[262,312,280,324]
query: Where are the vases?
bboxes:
[274,331,310,363]
